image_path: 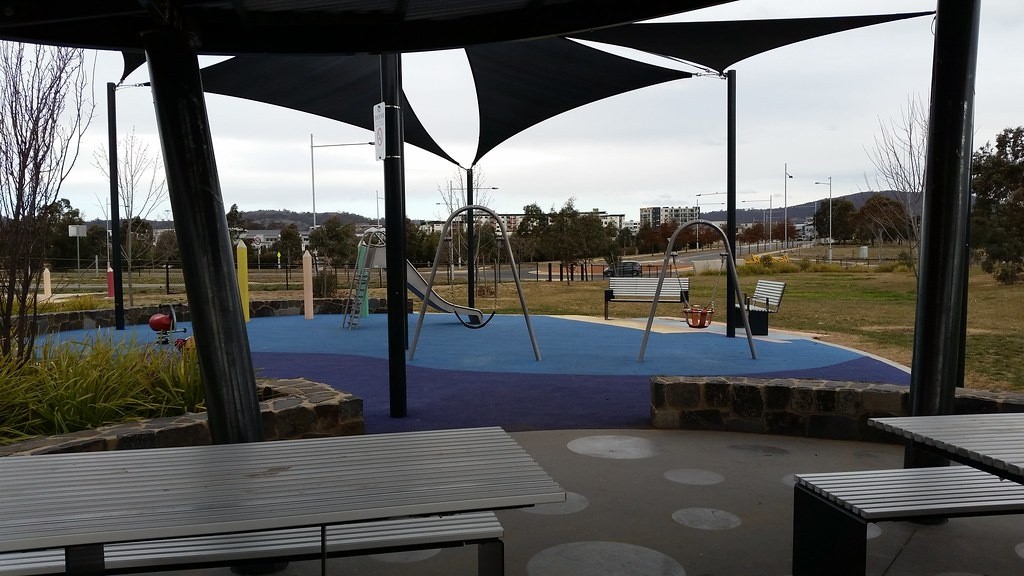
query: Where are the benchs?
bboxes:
[731,280,787,335]
[0,510,506,576]
[794,463,1024,576]
[602,276,690,319]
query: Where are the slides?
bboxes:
[381,238,484,325]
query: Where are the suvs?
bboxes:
[603,260,642,276]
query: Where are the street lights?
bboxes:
[436,198,461,269]
[784,162,793,254]
[696,199,725,249]
[449,180,498,280]
[106,197,130,268]
[311,134,375,275]
[815,177,832,262]
[742,195,772,250]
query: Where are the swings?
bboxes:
[671,253,729,328]
[449,217,499,329]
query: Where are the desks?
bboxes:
[0,421,565,576]
[867,411,1024,524]
